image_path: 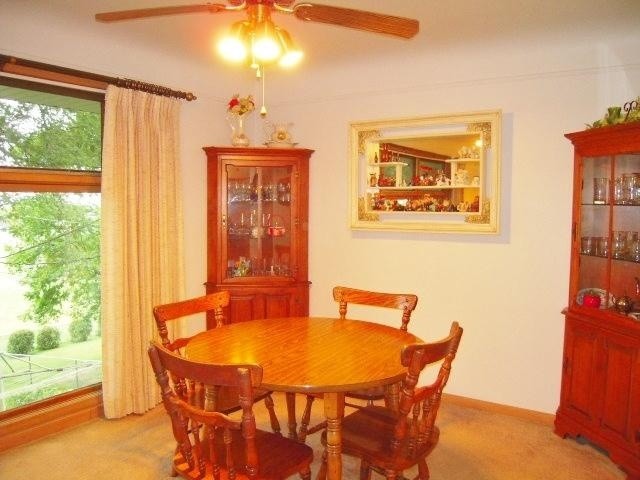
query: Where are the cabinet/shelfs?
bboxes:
[554,123,640,480]
[203,148,314,330]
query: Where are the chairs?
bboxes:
[316,321,463,479]
[147,339,313,480]
[153,292,282,436]
[299,286,417,441]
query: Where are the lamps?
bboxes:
[217,5,300,117]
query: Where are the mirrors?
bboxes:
[364,132,484,215]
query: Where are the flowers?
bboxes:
[226,92,256,117]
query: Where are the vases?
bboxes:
[226,116,250,147]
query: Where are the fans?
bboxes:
[96,0,420,39]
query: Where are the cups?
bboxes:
[594,177,607,202]
[228,182,290,203]
[581,237,596,255]
[610,174,640,205]
[228,210,271,229]
[597,237,607,257]
[612,231,640,262]
